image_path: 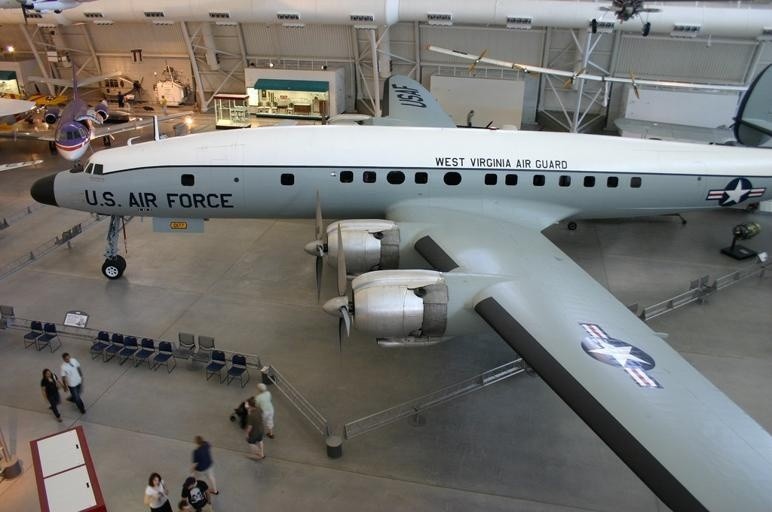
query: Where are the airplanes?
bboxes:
[586,0,664,37]
[26,69,772,511]
[0,58,194,172]
[0,0,99,23]
[0,88,68,132]
[1,160,45,171]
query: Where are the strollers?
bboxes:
[228,400,256,428]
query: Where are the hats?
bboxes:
[257,384,265,391]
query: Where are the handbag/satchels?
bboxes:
[53,373,61,389]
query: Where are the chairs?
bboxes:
[24,319,44,351]
[37,322,63,353]
[228,353,251,388]
[91,331,177,376]
[257,101,295,115]
[206,350,230,384]
[176,332,215,367]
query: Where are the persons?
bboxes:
[41,369,65,423]
[118,91,124,108]
[60,352,86,414]
[189,435,220,494]
[161,96,168,115]
[467,110,473,127]
[179,501,194,512]
[102,96,108,106]
[255,383,274,440]
[144,473,173,512]
[182,477,212,512]
[244,397,265,460]
